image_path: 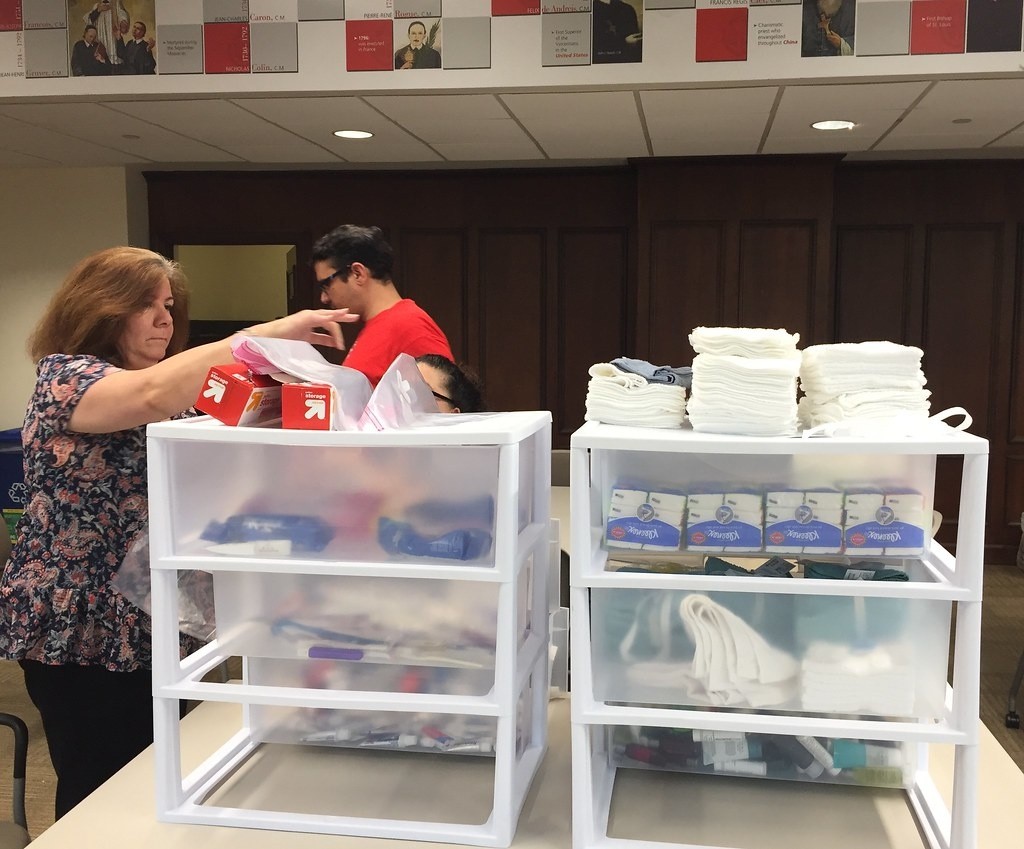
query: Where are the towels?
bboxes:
[679,594,800,708]
[798,341,933,434]
[583,356,691,429]
[686,325,803,438]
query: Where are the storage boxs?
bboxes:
[270,372,337,431]
[195,363,284,426]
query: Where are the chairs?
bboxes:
[0,713,32,849]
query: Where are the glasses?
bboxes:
[432,390,462,410]
[317,264,351,289]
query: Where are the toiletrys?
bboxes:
[285,690,528,757]
[204,539,292,559]
[611,726,905,788]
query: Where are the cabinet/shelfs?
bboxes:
[571,421,989,849]
[147,412,568,849]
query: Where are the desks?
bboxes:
[25,679,1024,849]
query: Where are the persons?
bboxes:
[411,354,491,418]
[0,247,359,825]
[312,222,456,390]
